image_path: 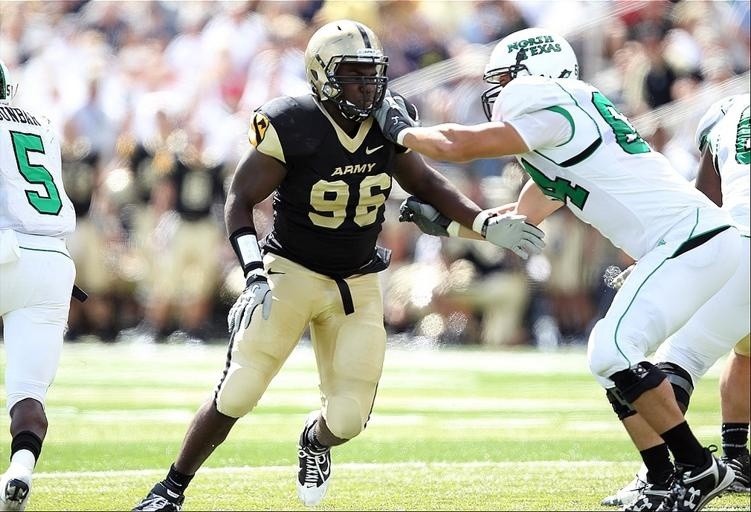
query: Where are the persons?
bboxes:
[599,88,751,508]
[133,19,547,510]
[1,59,83,510]
[367,27,741,510]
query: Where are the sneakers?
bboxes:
[721,450,751,492]
[0,465,31,512]
[619,475,674,512]
[296,409,333,506]
[654,448,735,512]
[599,468,648,505]
[130,480,186,512]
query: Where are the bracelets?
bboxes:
[244,272,269,288]
[473,207,492,234]
[445,218,464,242]
[395,127,411,147]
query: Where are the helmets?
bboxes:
[306,19,388,120]
[483,28,578,120]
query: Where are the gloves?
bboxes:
[398,196,461,239]
[372,87,418,147]
[225,278,274,335]
[475,212,548,264]
